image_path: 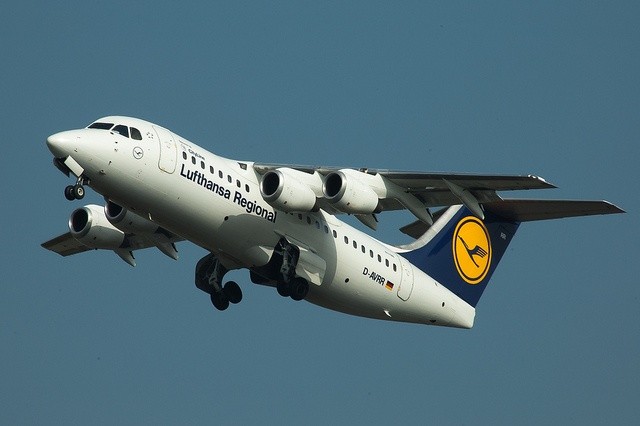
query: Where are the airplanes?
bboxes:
[40,115,625,330]
